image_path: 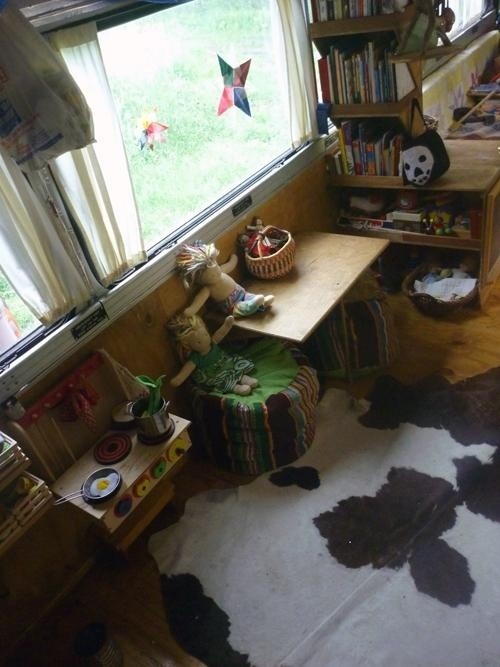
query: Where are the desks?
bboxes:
[204,228,391,345]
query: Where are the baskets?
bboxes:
[402,260,479,315]
[244,224,295,281]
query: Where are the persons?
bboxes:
[244,213,275,248]
[166,312,259,397]
[173,237,276,321]
[237,232,278,257]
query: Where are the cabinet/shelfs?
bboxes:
[330,139,499,310]
[309,1,424,187]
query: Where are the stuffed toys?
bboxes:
[421,211,470,238]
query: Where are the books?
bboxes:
[395,220,428,233]
[384,208,426,222]
[297,0,403,178]
[471,81,500,96]
[391,8,444,55]
[469,201,482,240]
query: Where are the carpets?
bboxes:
[146,366,500,667]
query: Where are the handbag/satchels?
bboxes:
[398,129,450,188]
